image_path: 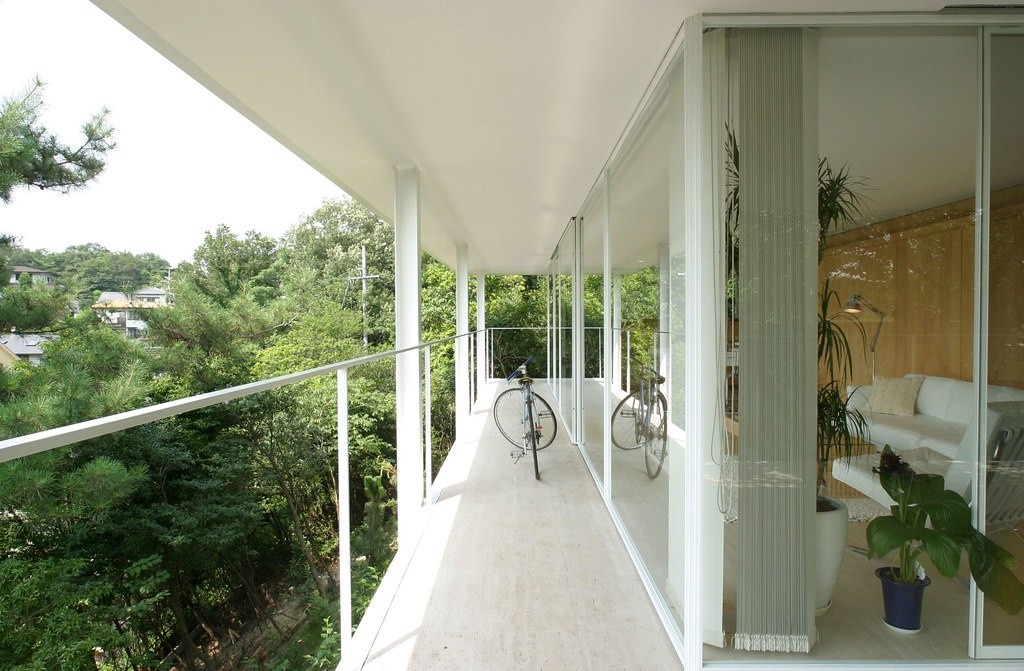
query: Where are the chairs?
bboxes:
[829,401,1024,604]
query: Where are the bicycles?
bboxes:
[492,355,558,481]
[611,355,668,480]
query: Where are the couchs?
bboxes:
[829,375,1024,459]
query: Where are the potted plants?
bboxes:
[863,444,1024,630]
[720,121,889,609]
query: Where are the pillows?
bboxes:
[860,374,926,417]
